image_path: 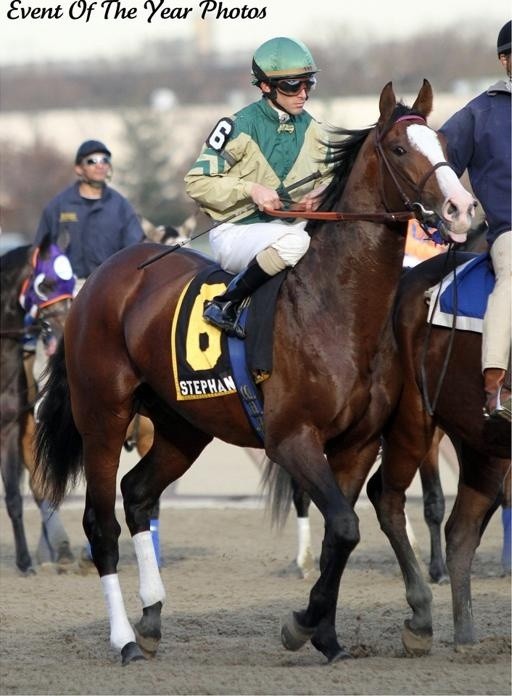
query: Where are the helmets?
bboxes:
[497,20,512,58]
[251,37,320,85]
[76,141,111,164]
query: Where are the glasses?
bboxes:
[271,78,316,96]
[85,158,111,164]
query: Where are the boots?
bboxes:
[185,255,273,339]
[485,369,512,410]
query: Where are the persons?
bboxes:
[30,140,147,297]
[437,18,512,423]
[183,37,336,340]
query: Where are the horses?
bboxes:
[366,230,512,648]
[256,430,450,585]
[18,214,198,573]
[0,230,77,577]
[30,76,477,666]
